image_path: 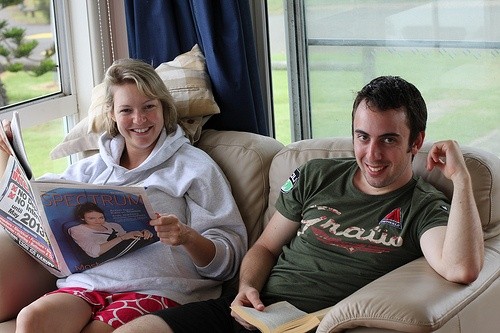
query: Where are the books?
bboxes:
[229,300,336,333]
[0,110,163,278]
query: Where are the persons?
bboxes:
[0,63,248,333]
[66,203,154,270]
[111,75,484,333]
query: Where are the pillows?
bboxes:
[50,114,212,160]
[87,43,220,135]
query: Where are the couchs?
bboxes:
[0,129,500,333]
